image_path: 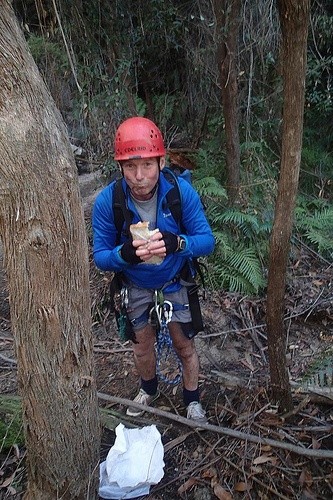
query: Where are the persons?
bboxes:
[92,117,216,431]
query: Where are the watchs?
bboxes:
[177,237,186,253]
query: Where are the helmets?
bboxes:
[113,117,166,160]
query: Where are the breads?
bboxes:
[130,221,164,264]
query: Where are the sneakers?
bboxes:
[126,388,160,417]
[186,401,209,430]
